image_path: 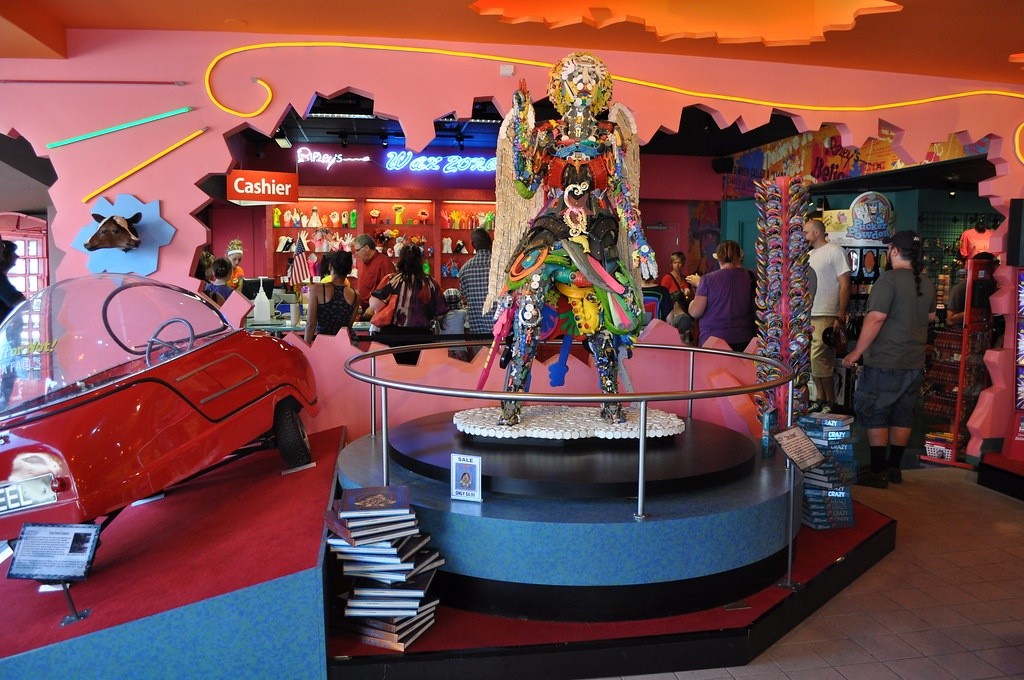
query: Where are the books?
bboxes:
[324,485,445,652]
[760,405,860,529]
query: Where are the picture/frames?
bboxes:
[450,453,482,503]
[5,521,102,583]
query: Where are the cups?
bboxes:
[290,304,300,327]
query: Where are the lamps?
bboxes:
[305,93,376,119]
[380,134,389,149]
[338,132,349,149]
[273,127,293,150]
[456,132,465,151]
[712,158,735,176]
[438,112,503,124]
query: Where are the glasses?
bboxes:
[355,244,366,252]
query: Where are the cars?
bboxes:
[0,271,319,556]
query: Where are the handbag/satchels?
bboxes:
[667,273,687,309]
[370,293,398,328]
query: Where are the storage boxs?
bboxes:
[924,430,963,462]
[277,304,304,316]
[282,293,297,304]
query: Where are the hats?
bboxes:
[444,288,462,309]
[882,230,920,250]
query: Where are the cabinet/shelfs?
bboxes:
[271,198,362,290]
[362,198,438,280]
[920,258,999,471]
[438,199,496,291]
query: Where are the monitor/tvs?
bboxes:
[240,278,274,299]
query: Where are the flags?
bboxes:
[290,236,311,286]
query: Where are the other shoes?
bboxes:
[888,466,902,484]
[821,401,838,414]
[807,399,826,412]
[852,465,888,488]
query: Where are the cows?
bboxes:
[84,212,142,251]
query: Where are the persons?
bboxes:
[225,234,244,289]
[688,239,756,352]
[946,251,999,325]
[666,291,694,345]
[304,249,360,346]
[961,214,995,271]
[662,251,694,322]
[498,47,658,426]
[368,227,493,360]
[0,239,27,413]
[204,257,232,306]
[198,252,216,285]
[841,229,937,487]
[320,253,350,287]
[354,234,396,322]
[641,258,674,323]
[804,219,851,414]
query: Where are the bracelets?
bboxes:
[836,316,845,323]
